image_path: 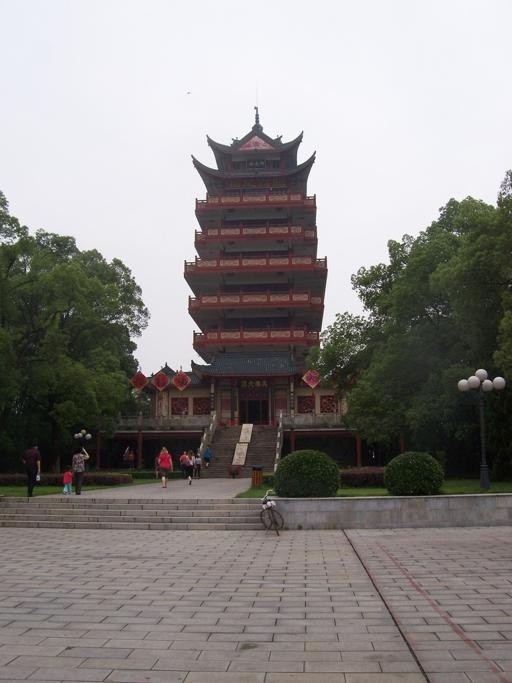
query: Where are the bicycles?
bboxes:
[254,488,285,539]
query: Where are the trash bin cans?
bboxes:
[252,466,263,488]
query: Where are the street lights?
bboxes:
[457,369,507,491]
[72,428,93,453]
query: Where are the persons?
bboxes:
[21,440,42,497]
[128,449,136,470]
[72,445,91,495]
[154,444,215,488]
[62,464,73,495]
